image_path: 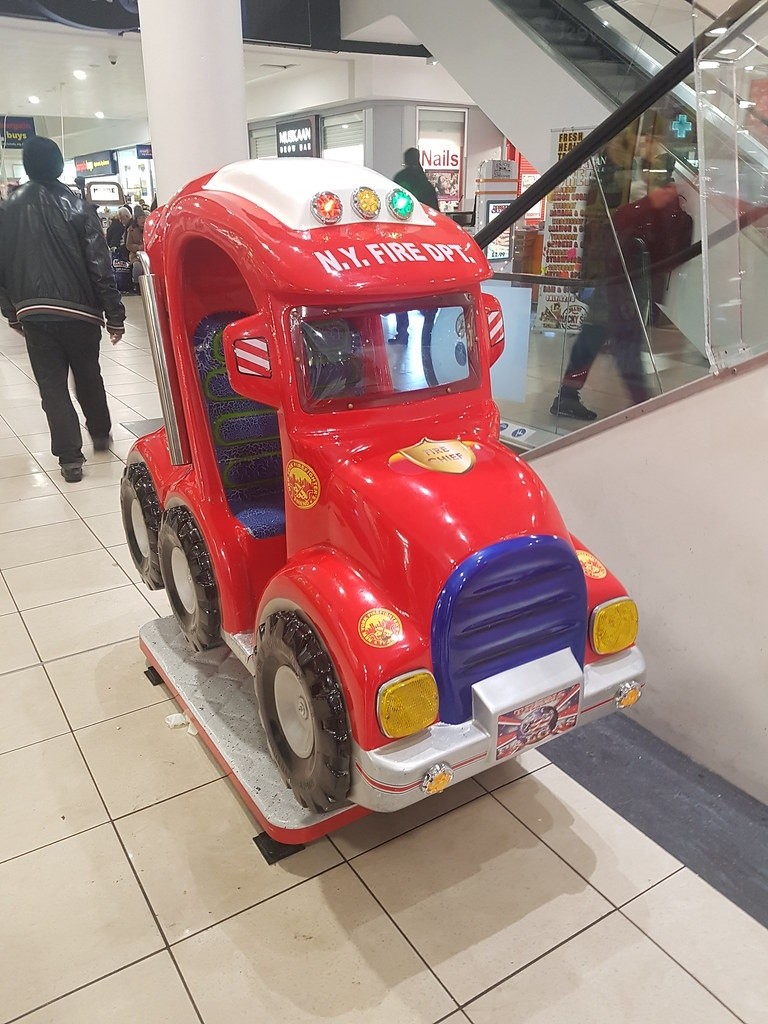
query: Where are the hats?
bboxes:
[134,206,146,218]
[23,137,64,181]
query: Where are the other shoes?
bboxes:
[92,434,112,450]
[61,467,82,483]
[133,284,140,293]
[551,396,598,421]
[387,336,408,345]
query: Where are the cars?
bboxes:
[118,153,643,816]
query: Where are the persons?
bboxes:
[387,148,440,346]
[550,106,678,420]
[0,137,127,484]
[106,193,158,296]
